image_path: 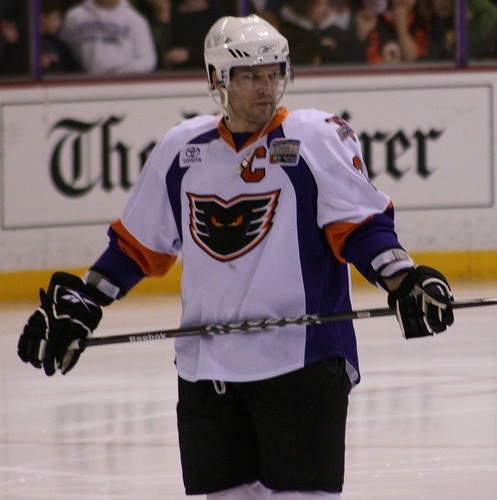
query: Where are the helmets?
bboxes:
[205,13,290,90]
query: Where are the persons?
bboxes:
[1,1,497,78]
[17,14,454,500]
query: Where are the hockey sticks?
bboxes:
[67,297,497,345]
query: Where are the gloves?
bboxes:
[387,265,455,339]
[16,273,116,376]
